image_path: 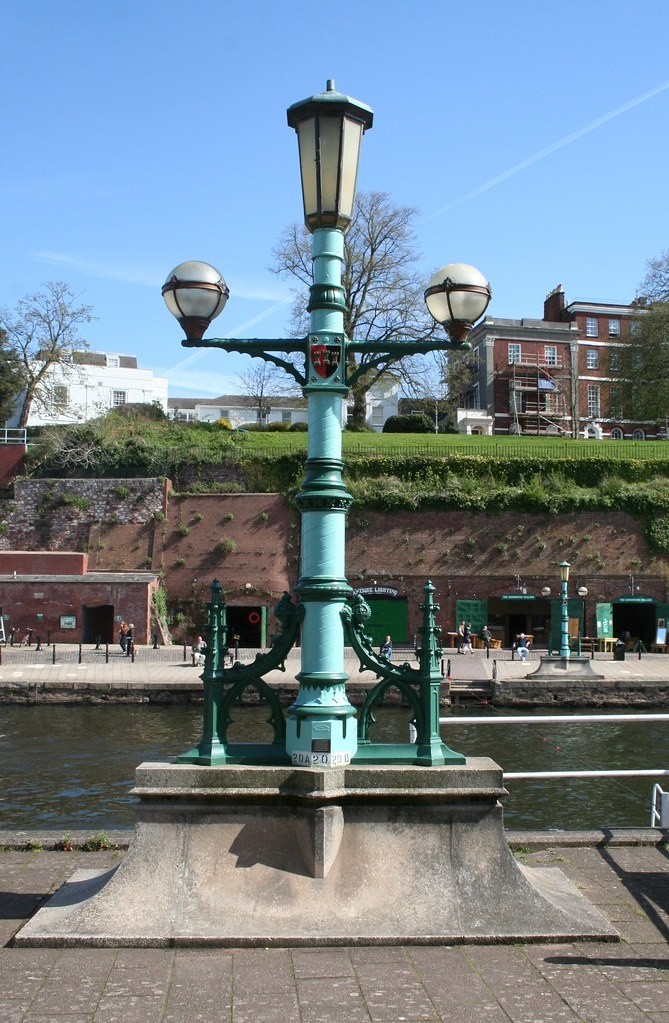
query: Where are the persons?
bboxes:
[378,636,393,661]
[192,636,207,654]
[479,626,491,649]
[457,620,475,654]
[514,633,531,661]
[118,620,136,657]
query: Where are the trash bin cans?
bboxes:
[614,639,626,661]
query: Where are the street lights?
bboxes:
[525,560,605,680]
[12,77,626,947]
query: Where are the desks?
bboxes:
[570,637,598,652]
[600,637,618,653]
[447,633,478,648]
[516,634,534,650]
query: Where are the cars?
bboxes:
[238,429,249,432]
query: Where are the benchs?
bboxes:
[190,653,234,666]
[651,643,667,653]
[379,651,418,662]
[581,642,598,651]
[456,407,496,436]
